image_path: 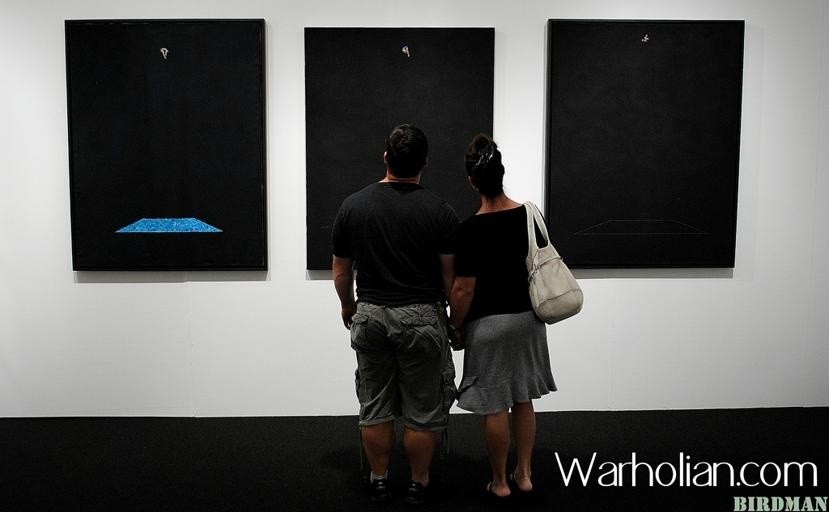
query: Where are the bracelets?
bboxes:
[449,325,461,334]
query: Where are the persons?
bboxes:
[449,133,559,500]
[331,124,461,506]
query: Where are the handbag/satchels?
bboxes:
[526,242,583,325]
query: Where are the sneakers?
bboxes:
[407,471,433,507]
[366,470,390,503]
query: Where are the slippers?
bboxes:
[487,480,512,499]
[509,471,533,494]
